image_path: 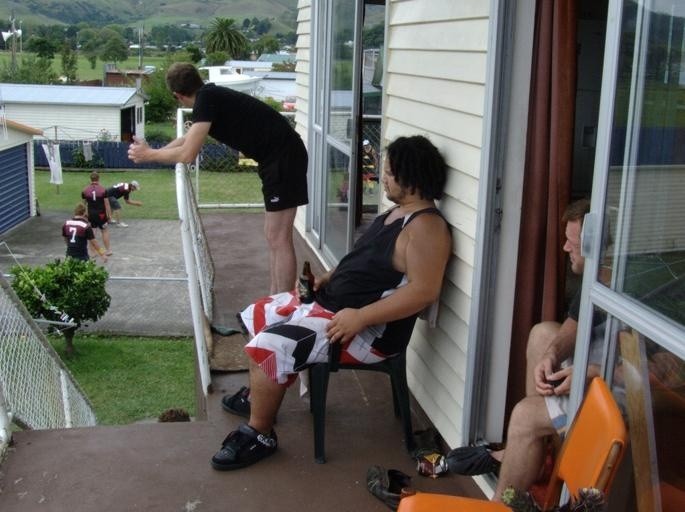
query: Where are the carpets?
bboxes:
[209,322,250,372]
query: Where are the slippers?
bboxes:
[446,442,502,475]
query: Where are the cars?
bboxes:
[199,67,258,83]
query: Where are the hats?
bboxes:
[129,179,141,191]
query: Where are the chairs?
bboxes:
[297,310,420,463]
[393,377,625,512]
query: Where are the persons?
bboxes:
[211,134,453,470]
[447,201,659,506]
[361,139,378,197]
[81,171,113,259]
[105,179,143,228]
[62,203,108,265]
[126,61,309,299]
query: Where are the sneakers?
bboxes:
[219,386,254,418]
[364,465,414,507]
[209,426,279,472]
[116,223,128,228]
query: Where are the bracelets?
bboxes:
[97,247,106,255]
